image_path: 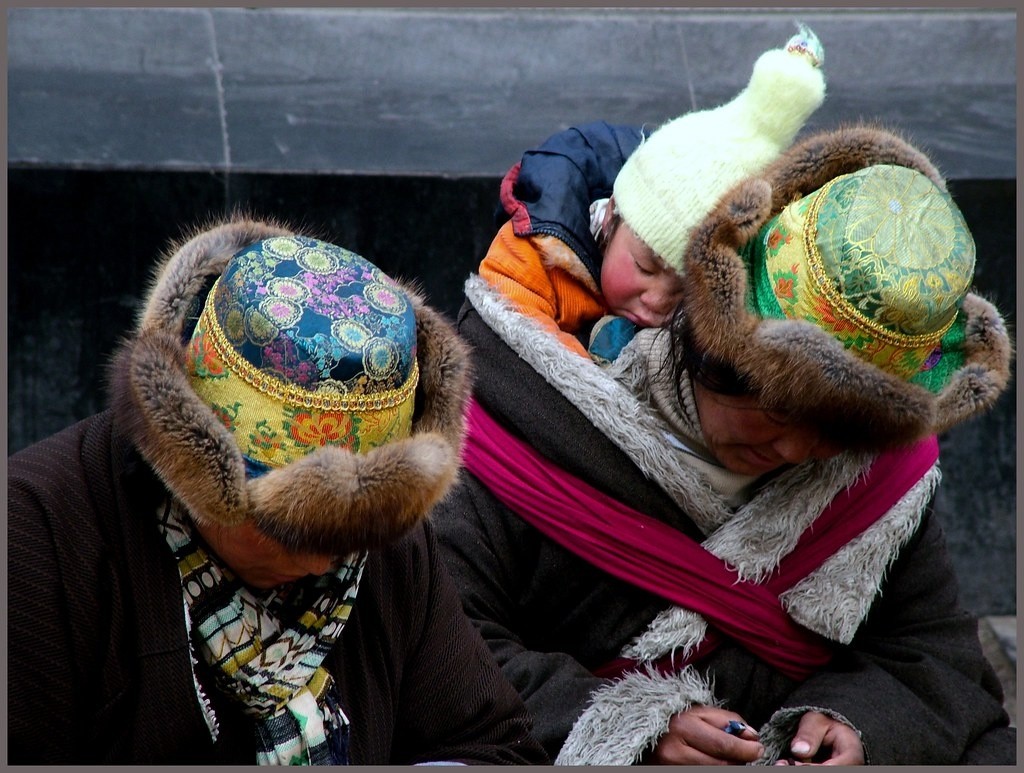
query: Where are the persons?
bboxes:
[478,34,826,363]
[426,131,1016,765]
[5,220,543,767]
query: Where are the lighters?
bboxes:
[722,720,746,737]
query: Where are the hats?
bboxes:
[682,127,1009,445]
[105,211,477,554]
[614,18,827,279]
